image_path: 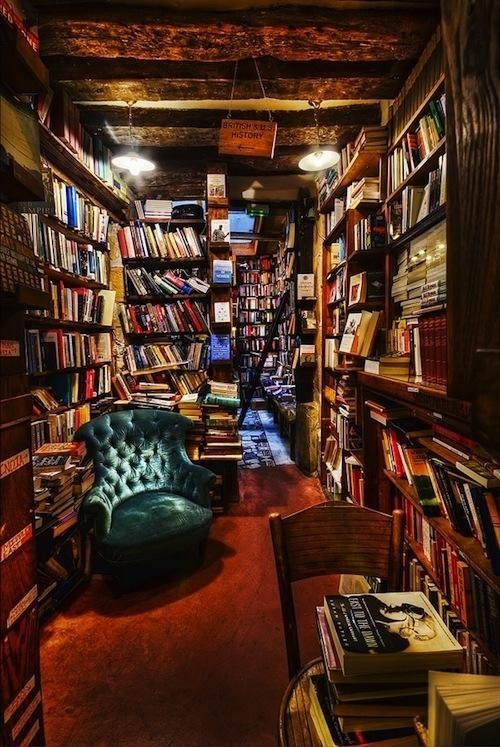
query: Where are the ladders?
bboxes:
[238,291,291,429]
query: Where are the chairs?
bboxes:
[268,499,405,684]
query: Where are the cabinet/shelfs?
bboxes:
[0,0,500,669]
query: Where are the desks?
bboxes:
[280,654,429,747]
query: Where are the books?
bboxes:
[1,76,500,747]
